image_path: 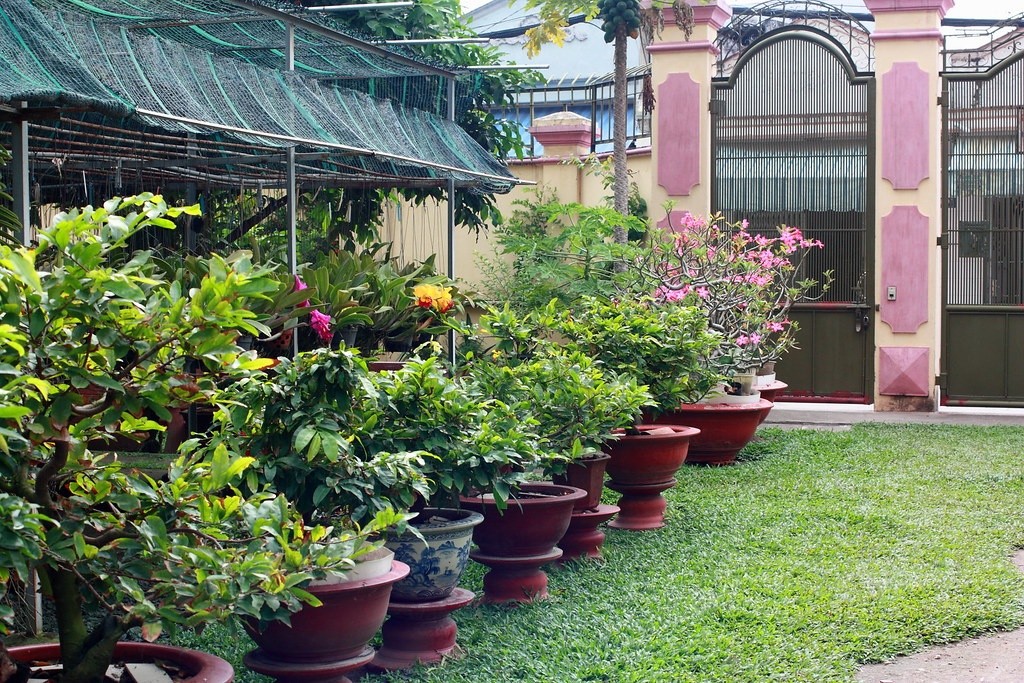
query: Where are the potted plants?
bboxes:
[438,324,608,608]
[555,294,734,528]
[523,301,660,560]
[372,343,556,671]
[494,201,774,466]
[221,346,441,683]
[0,186,418,683]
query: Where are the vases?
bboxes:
[714,378,788,427]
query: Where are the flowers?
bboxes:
[648,202,834,368]
[291,275,336,344]
[409,283,457,321]
